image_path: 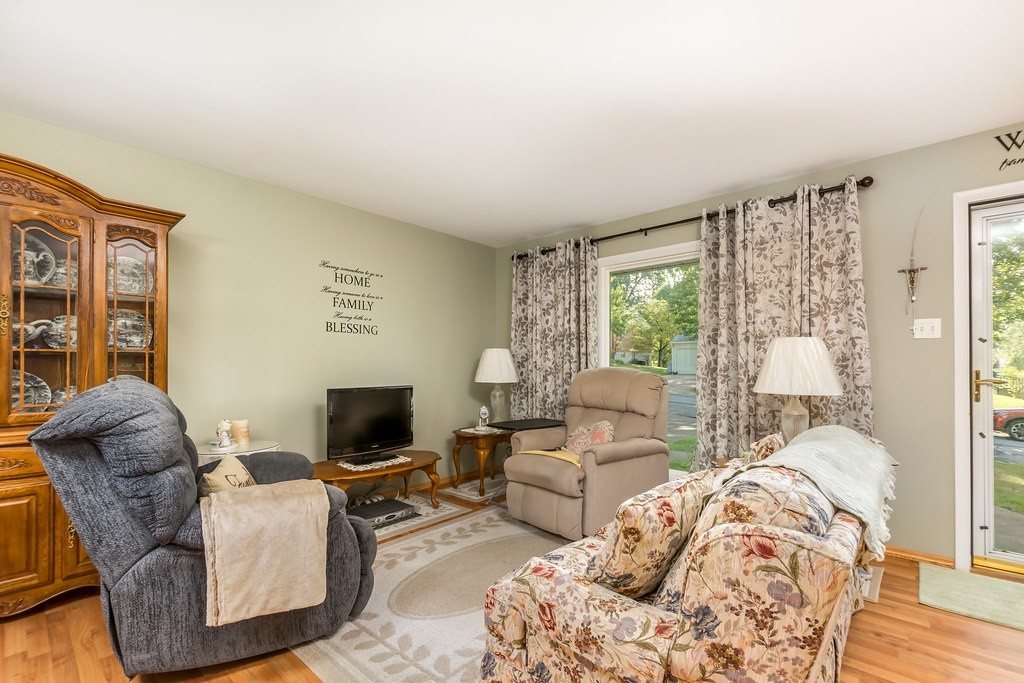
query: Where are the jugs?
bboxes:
[12,320,53,348]
[11,250,55,285]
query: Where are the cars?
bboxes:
[994,407,1024,442]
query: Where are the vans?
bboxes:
[614,352,632,361]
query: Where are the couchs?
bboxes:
[26,380,378,680]
[503,366,670,542]
[478,425,898,683]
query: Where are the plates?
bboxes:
[12,228,55,282]
[107,308,153,351]
[12,369,52,413]
[43,258,79,289]
[107,374,146,383]
[48,385,77,412]
[106,255,154,293]
[42,314,77,349]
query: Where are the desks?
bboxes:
[310,449,443,509]
[197,441,281,468]
[452,415,514,498]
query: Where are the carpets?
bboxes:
[288,500,574,683]
[375,491,472,542]
[436,473,506,503]
[917,561,1024,632]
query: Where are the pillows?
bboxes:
[582,455,748,599]
[197,452,257,498]
[746,431,786,464]
[565,421,616,454]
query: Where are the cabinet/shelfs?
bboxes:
[0,153,186,619]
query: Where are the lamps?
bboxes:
[753,337,844,443]
[475,348,519,423]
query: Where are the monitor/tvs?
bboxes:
[326,385,414,466]
